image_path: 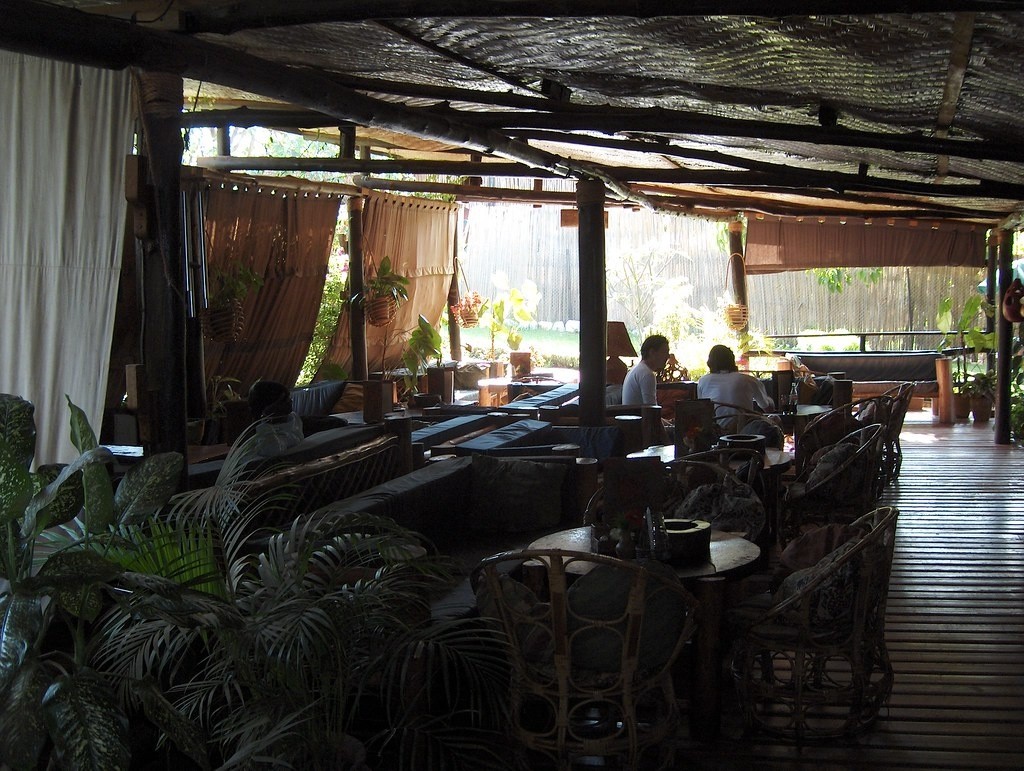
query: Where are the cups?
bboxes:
[780,394,788,415]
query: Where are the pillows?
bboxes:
[470,451,570,536]
[290,379,347,418]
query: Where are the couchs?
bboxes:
[185,379,696,770]
[777,353,953,423]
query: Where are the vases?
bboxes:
[615,533,635,560]
[461,313,477,325]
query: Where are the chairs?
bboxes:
[483,381,917,771]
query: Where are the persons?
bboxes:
[248,382,303,456]
[621,335,676,426]
[697,345,782,431]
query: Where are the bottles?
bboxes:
[788,383,798,413]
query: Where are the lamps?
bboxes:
[607,321,638,383]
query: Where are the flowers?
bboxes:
[450,292,481,315]
[610,509,646,536]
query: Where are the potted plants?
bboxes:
[725,304,741,324]
[365,312,443,424]
[205,259,264,344]
[477,287,534,376]
[952,371,997,422]
[349,255,409,326]
